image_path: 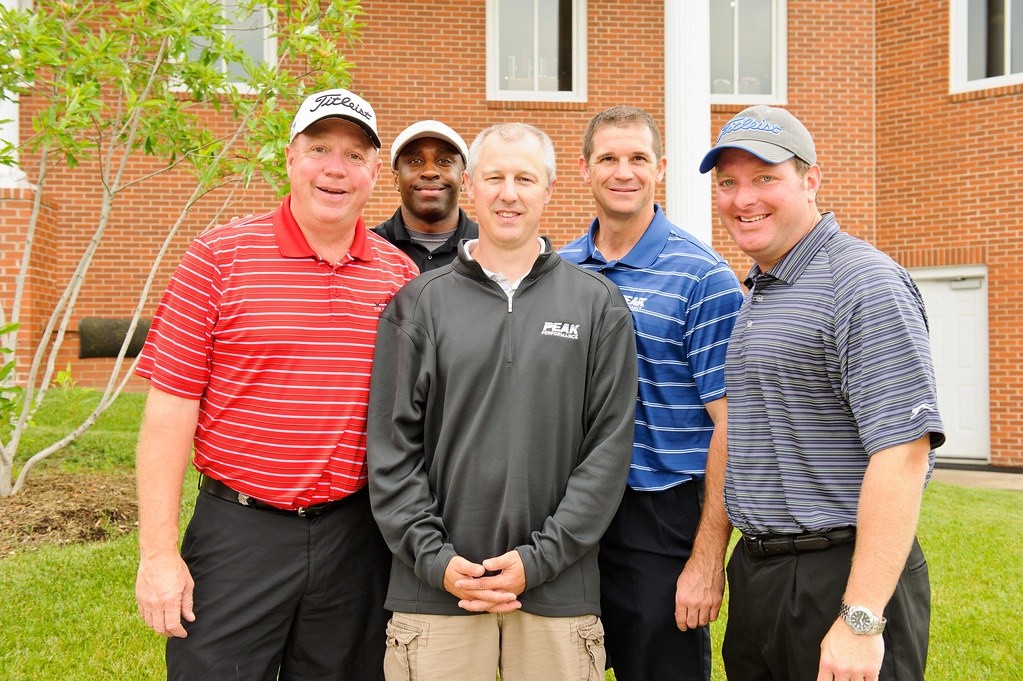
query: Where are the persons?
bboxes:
[366,106,745,681]
[135,88,421,681]
[698,105,947,681]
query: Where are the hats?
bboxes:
[698,106,816,174]
[290,89,380,156]
[389,121,468,170]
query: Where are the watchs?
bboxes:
[838,602,888,635]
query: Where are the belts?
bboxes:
[741,527,854,557]
[200,475,340,518]
